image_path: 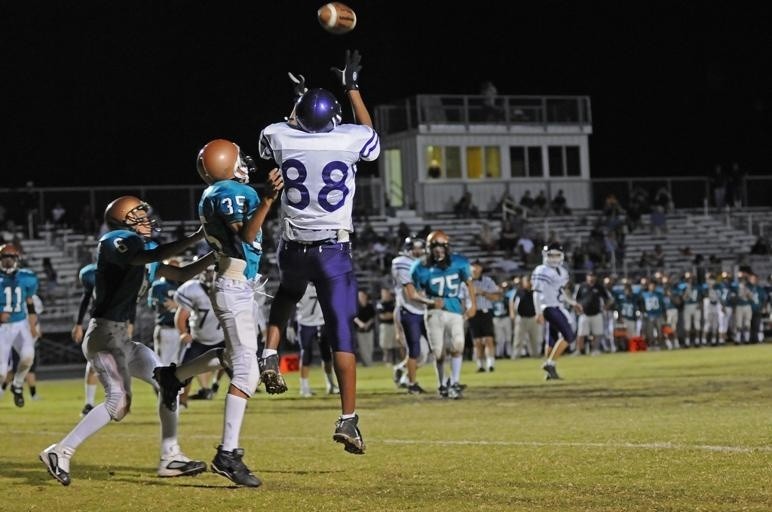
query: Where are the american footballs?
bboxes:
[317,3,357,34]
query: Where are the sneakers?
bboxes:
[477,365,495,374]
[39,443,71,487]
[157,454,207,479]
[152,362,184,413]
[393,365,468,401]
[10,383,24,408]
[333,413,367,456]
[257,354,289,396]
[210,444,262,489]
[541,362,559,379]
[187,388,213,400]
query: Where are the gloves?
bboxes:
[330,49,365,94]
[286,55,310,97]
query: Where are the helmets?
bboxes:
[196,139,251,187]
[104,195,154,240]
[1,244,21,275]
[541,241,564,268]
[405,231,427,262]
[425,230,450,262]
[294,87,343,134]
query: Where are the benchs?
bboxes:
[1,207,768,366]
